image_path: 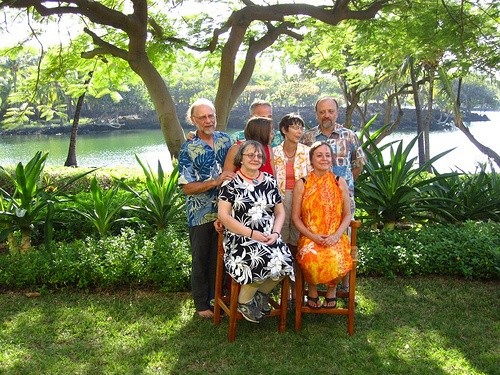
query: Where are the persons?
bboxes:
[178,97,364,323]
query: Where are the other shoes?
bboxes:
[197,308,225,317]
[222,295,231,302]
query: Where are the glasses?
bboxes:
[193,113,216,121]
[241,153,265,159]
[287,125,306,131]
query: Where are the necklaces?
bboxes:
[238,167,261,184]
[283,145,295,158]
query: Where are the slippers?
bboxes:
[308,295,322,309]
[323,297,337,308]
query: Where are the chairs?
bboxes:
[213,215,361,343]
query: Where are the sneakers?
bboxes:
[237,299,264,324]
[255,291,269,312]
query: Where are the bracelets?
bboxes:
[250,229,253,238]
[273,232,280,238]
